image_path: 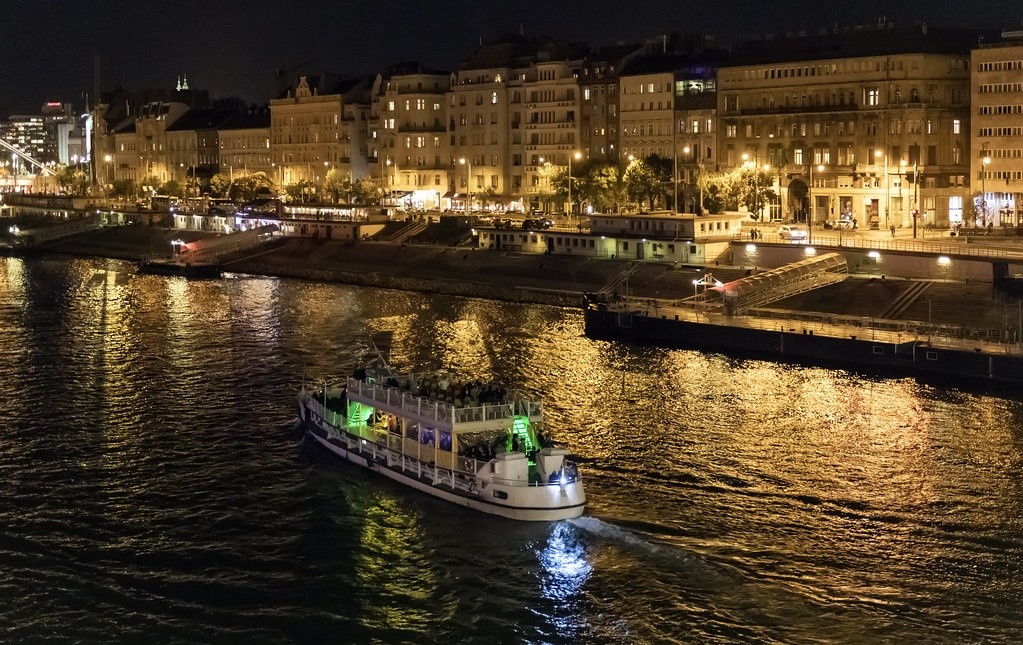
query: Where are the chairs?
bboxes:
[422,382,504,407]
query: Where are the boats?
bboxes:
[136,239,223,279]
[295,333,590,522]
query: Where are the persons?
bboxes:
[367,409,401,435]
[468,429,562,462]
[928,220,932,232]
[750,228,758,240]
[353,364,366,382]
[384,373,509,408]
[853,219,857,226]
[891,225,895,237]
[315,211,334,221]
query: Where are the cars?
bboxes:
[778,226,806,241]
[444,208,554,231]
[832,219,859,231]
[770,219,784,224]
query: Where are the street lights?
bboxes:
[874,149,890,230]
[323,162,335,171]
[105,155,112,202]
[742,145,759,225]
[180,163,196,196]
[566,151,582,224]
[459,157,469,212]
[981,157,991,228]
[44,171,50,196]
[271,162,282,219]
[540,157,548,211]
[673,146,692,214]
[379,157,391,201]
[809,163,827,243]
